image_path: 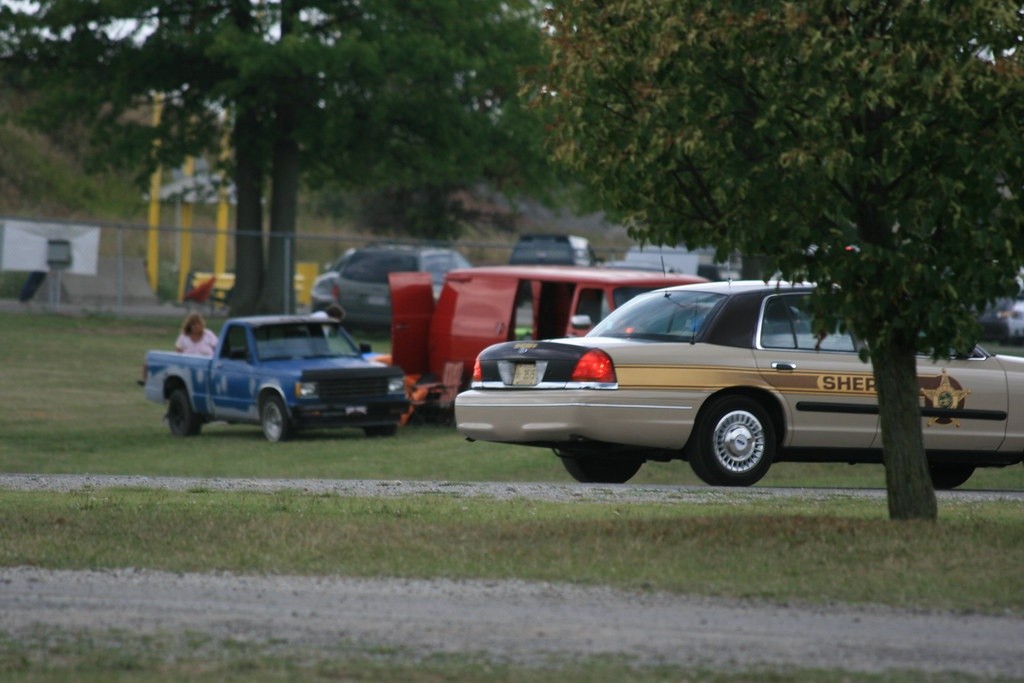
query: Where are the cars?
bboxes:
[1009,296,1024,340]
[976,299,1011,347]
[306,248,480,334]
[451,279,1022,487]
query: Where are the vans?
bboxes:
[387,266,712,416]
[507,232,607,276]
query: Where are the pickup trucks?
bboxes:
[144,314,410,445]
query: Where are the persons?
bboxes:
[176,313,219,359]
